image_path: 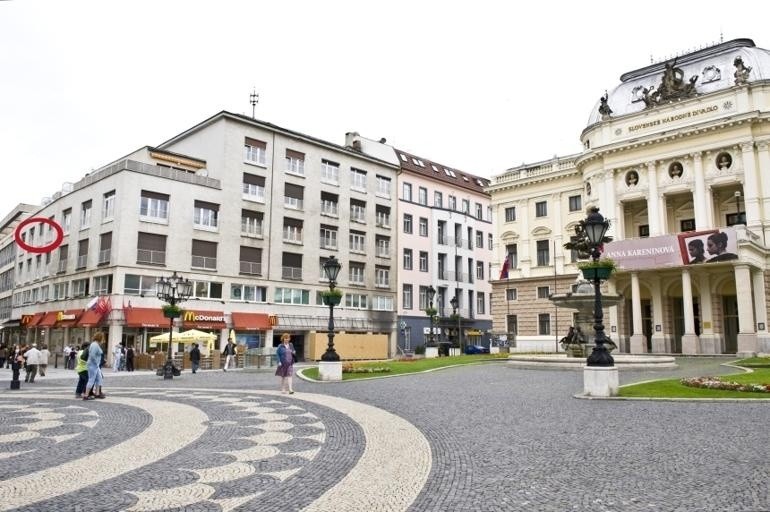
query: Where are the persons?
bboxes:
[221,337,238,371]
[1,330,134,401]
[274,332,298,395]
[558,326,574,351]
[189,343,201,374]
[571,327,587,353]
[687,239,707,264]
[596,88,614,120]
[664,56,680,84]
[705,230,739,262]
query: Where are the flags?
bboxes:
[497,254,512,279]
[84,295,133,322]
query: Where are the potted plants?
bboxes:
[577,257,617,280]
[319,290,343,305]
[160,304,183,319]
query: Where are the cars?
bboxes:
[466,344,489,354]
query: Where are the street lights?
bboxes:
[154,272,193,380]
[320,255,343,360]
[450,297,459,316]
[736,191,742,222]
[581,205,617,365]
[248,87,261,118]
[425,285,437,345]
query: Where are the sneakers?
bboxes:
[76,392,105,400]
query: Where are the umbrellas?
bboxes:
[230,328,238,355]
[205,331,216,359]
[150,331,180,344]
[174,327,217,345]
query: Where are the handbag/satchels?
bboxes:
[81,348,88,360]
[292,353,298,362]
